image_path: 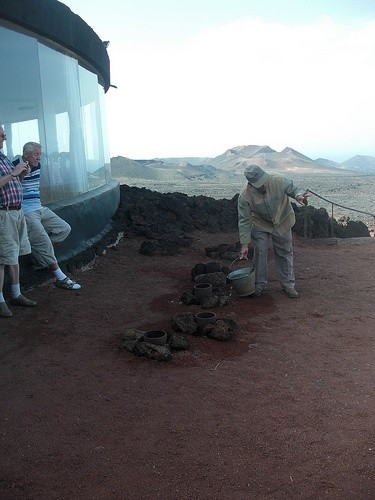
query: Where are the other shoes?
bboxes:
[0,302,13,318]
[12,296,37,307]
[251,287,267,297]
[282,285,298,297]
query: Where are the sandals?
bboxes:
[55,277,81,290]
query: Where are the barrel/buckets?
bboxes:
[227,257,256,297]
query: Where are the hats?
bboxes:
[244,164,270,188]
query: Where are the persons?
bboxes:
[0,125,37,316]
[238,164,309,298]
[12,142,81,290]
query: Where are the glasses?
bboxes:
[0,133,6,137]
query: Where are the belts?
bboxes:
[0,205,21,210]
